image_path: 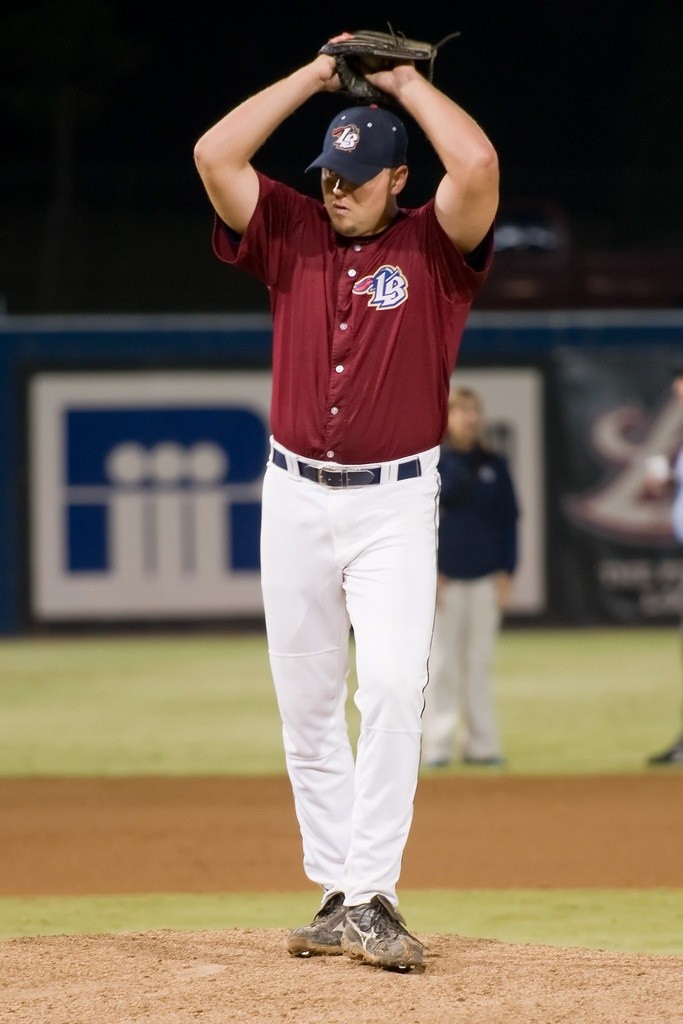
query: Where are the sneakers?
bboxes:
[288,891,349,956]
[340,894,430,969]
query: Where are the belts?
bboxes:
[272,448,421,489]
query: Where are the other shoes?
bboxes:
[422,752,451,766]
[464,746,500,764]
[648,741,683,766]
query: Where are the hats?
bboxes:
[304,105,408,183]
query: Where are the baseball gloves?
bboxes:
[317,18,460,99]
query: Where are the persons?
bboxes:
[420,386,519,770]
[194,32,499,968]
[647,369,683,766]
